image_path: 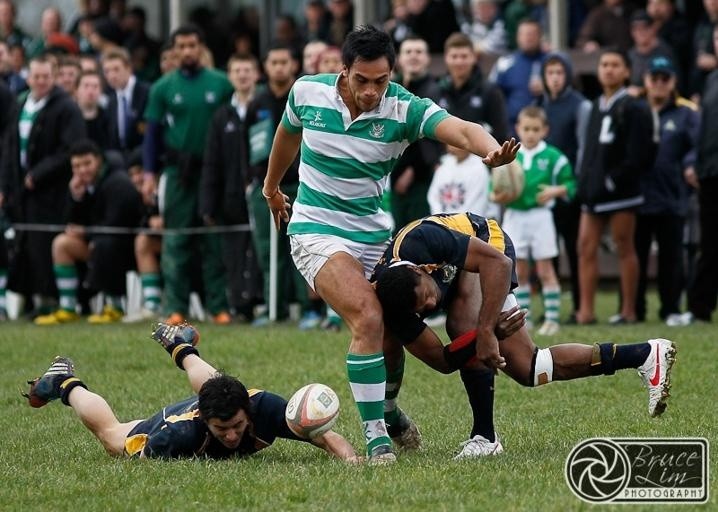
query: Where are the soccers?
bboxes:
[285,383,341,438]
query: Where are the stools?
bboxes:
[82,270,206,323]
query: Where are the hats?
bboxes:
[642,55,676,77]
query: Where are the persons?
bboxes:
[365,210,680,463]
[260,23,526,470]
[18,319,370,468]
[1,0,717,340]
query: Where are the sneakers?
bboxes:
[537,319,560,336]
[637,338,677,418]
[366,445,397,464]
[28,355,75,408]
[151,324,199,350]
[34,304,232,323]
[387,409,424,452]
[451,434,504,459]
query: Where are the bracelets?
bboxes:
[263,186,279,200]
